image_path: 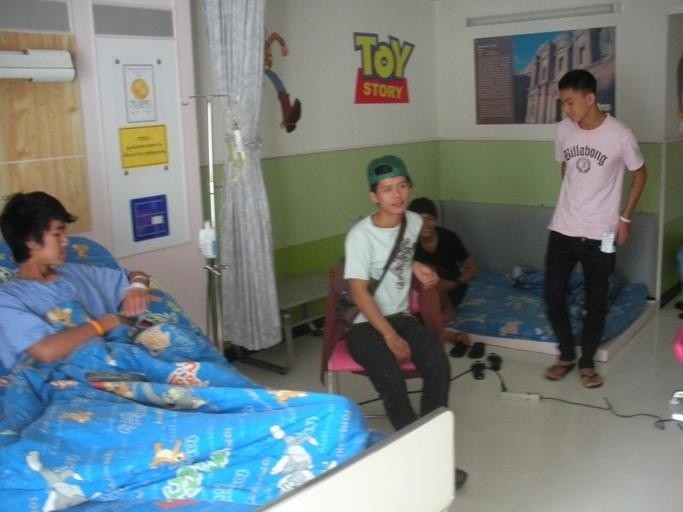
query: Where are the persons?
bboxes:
[542,70,648,388]
[0,191,149,372]
[342,155,450,430]
[405,198,477,346]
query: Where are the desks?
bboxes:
[228,268,332,376]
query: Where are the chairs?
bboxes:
[669,319,683,421]
[320,252,441,398]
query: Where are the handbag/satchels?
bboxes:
[332,275,381,324]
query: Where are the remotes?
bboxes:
[85,370,149,383]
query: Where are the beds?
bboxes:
[0,232,469,512]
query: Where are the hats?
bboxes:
[366,154,415,189]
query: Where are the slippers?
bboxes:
[448,337,502,381]
[578,362,605,390]
[543,356,577,383]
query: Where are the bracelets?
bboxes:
[130,275,151,282]
[619,216,632,225]
[90,321,104,336]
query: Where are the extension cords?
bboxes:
[498,390,540,402]
